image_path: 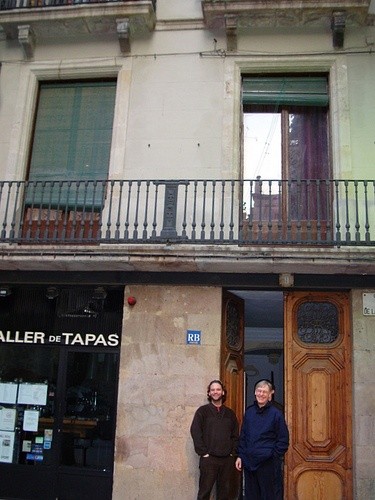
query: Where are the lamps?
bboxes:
[45,287,59,299]
[0,288,12,297]
[92,286,107,300]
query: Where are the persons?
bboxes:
[236,379,290,499]
[190,379,241,500]
